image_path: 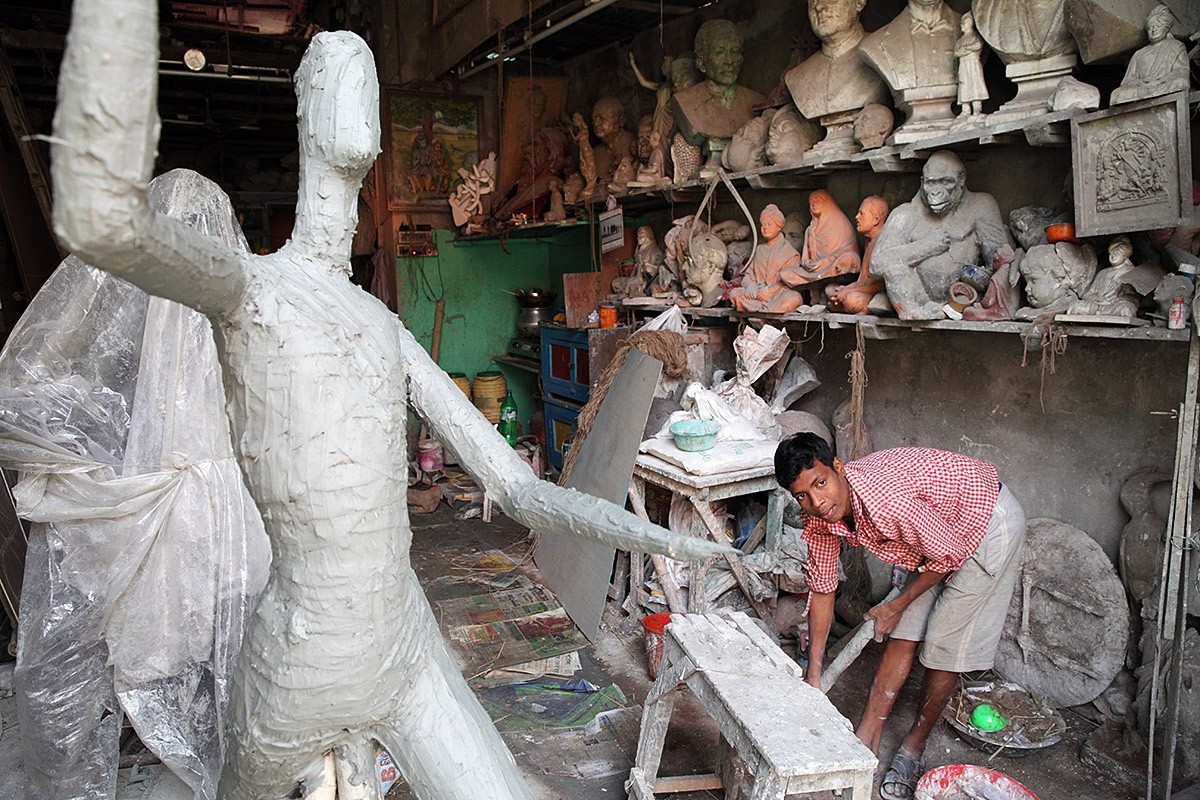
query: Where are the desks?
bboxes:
[629,450,789,618]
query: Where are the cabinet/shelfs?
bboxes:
[457,0,1199,345]
[537,321,592,467]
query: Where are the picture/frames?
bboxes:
[380,86,486,213]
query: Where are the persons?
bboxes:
[60,3,784,799]
[772,432,1030,800]
[421,1,1200,355]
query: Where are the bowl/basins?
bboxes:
[940,681,1067,758]
[669,419,722,452]
[914,764,1041,800]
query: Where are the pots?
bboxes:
[515,287,557,308]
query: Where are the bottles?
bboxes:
[499,390,518,448]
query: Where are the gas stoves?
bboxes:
[508,338,541,361]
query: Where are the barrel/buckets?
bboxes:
[417,438,443,472]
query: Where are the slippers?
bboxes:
[879,752,927,800]
